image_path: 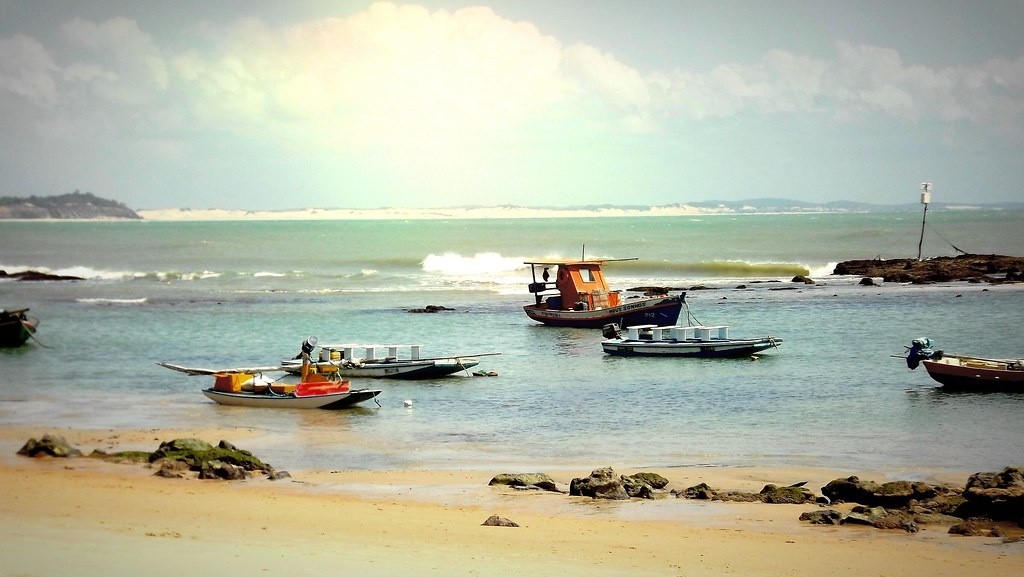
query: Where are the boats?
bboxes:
[279,345,478,383]
[0,308,37,348]
[905,338,1020,390]
[522,253,686,328]
[204,367,382,410]
[600,323,782,361]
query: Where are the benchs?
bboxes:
[375,345,424,361]
[650,326,681,340]
[698,326,729,341]
[318,344,356,361]
[340,345,385,360]
[627,325,657,340]
[675,326,705,341]
